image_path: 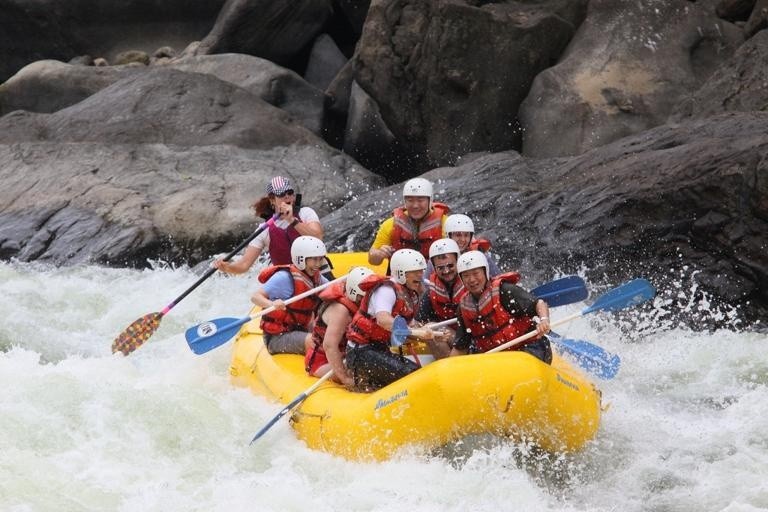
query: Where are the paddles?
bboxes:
[544,333,619,380]
[484,278,656,353]
[111,212,284,355]
[249,359,347,444]
[391,315,443,347]
[422,276,588,328]
[185,274,349,355]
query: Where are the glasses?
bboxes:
[276,190,294,198]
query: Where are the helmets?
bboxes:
[291,178,490,302]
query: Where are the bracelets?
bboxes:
[540,317,550,321]
[290,217,299,227]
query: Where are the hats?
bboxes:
[266,176,293,195]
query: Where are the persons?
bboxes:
[247,233,330,357]
[447,250,554,366]
[367,177,449,278]
[424,211,502,281]
[211,175,336,283]
[346,247,443,387]
[415,238,463,360]
[304,266,378,387]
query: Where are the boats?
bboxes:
[229,252,604,463]
